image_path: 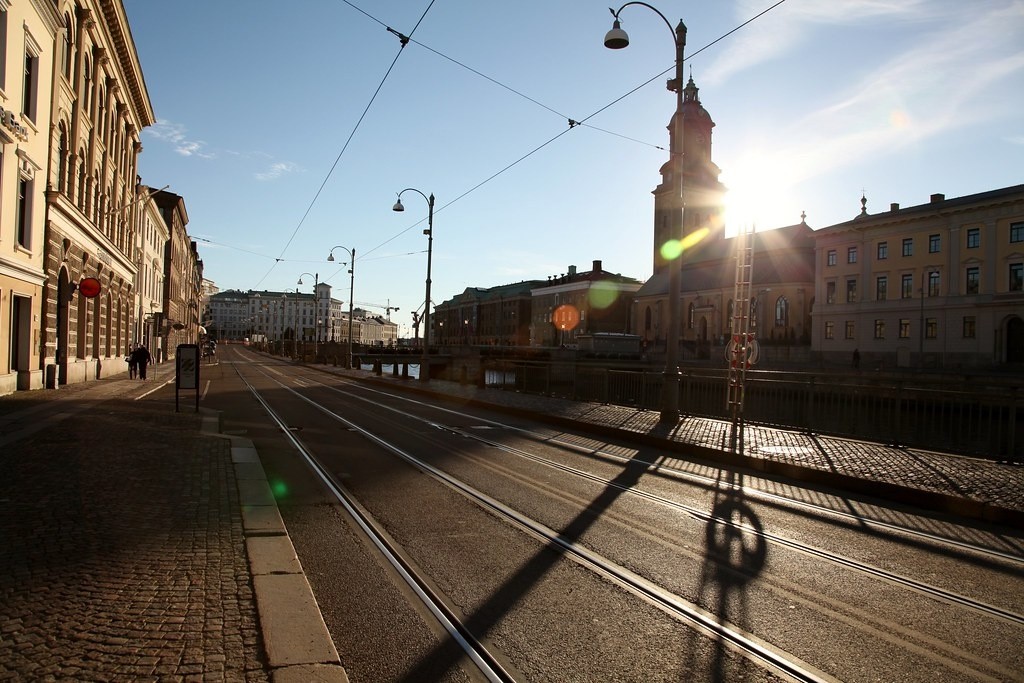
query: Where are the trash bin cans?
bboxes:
[47,364,59,389]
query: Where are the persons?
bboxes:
[853,348,861,368]
[129,343,140,380]
[136,342,150,381]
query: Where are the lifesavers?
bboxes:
[728,333,757,368]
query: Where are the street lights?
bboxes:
[329,249,357,365]
[493,294,504,358]
[688,294,703,363]
[391,188,435,346]
[653,298,662,353]
[921,264,945,374]
[628,298,640,335]
[749,288,771,337]
[240,293,287,358]
[297,272,320,360]
[560,323,566,349]
[1000,312,1018,375]
[604,2,687,363]
